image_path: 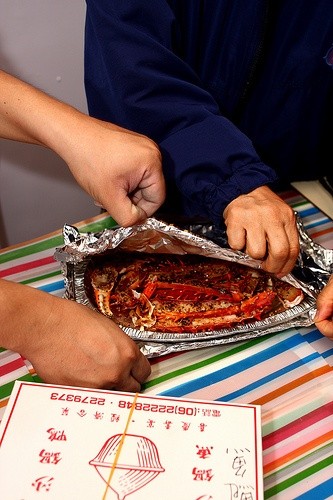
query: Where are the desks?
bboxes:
[0,179,333,500]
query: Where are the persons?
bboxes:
[0,66,167,392]
[83,0,333,341]
